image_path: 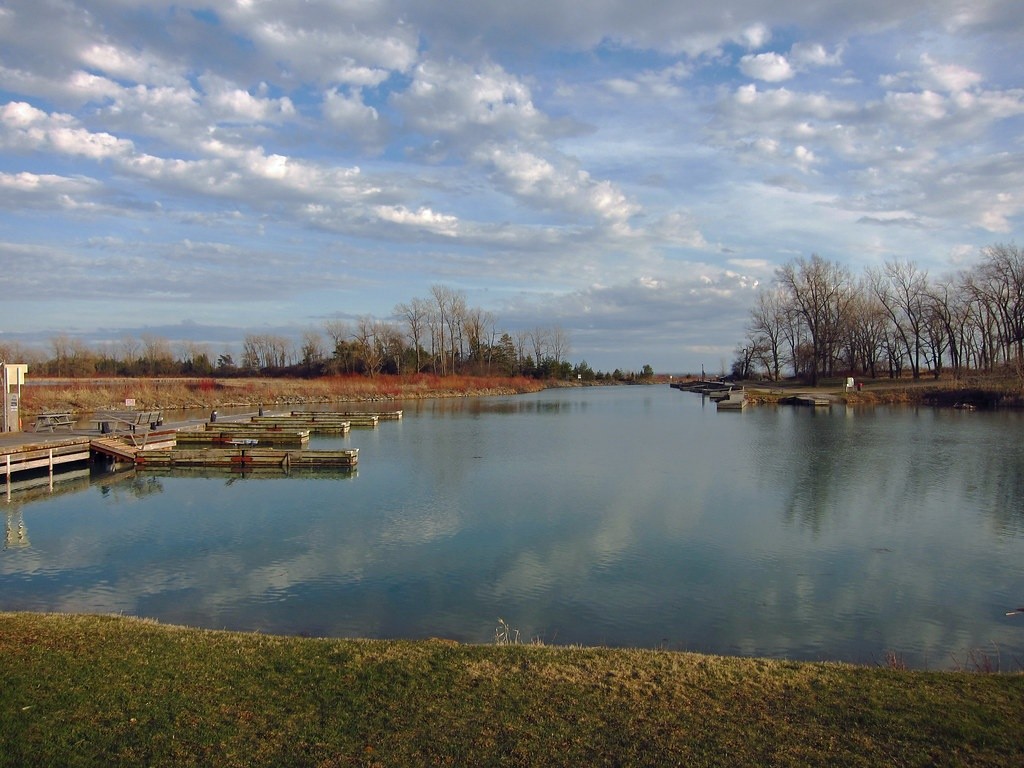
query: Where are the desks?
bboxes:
[33,414,73,433]
[89,420,116,433]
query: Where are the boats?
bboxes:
[680,383,729,394]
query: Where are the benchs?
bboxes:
[43,421,77,427]
[96,411,144,430]
[29,422,52,426]
[126,412,163,434]
